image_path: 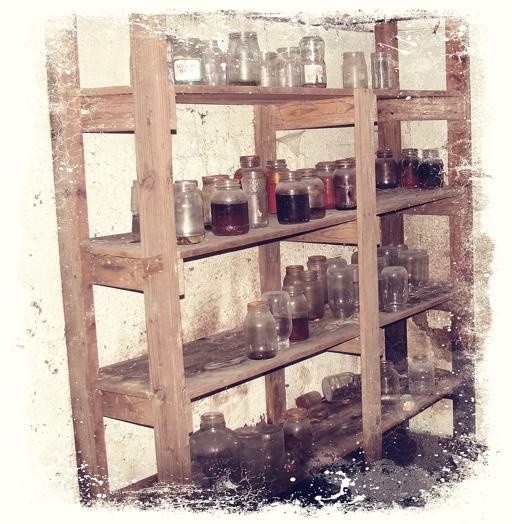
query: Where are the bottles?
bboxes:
[162,30,392,89]
[421,148,444,189]
[380,266,407,312]
[246,447,276,478]
[401,149,419,187]
[286,407,313,462]
[380,361,400,405]
[248,253,355,361]
[172,152,356,241]
[258,424,286,469]
[375,148,398,189]
[408,353,435,393]
[192,411,241,487]
[130,181,142,244]
[378,246,400,276]
[323,371,361,403]
[403,249,428,287]
[235,425,261,463]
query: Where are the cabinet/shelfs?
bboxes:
[46,11,475,510]
